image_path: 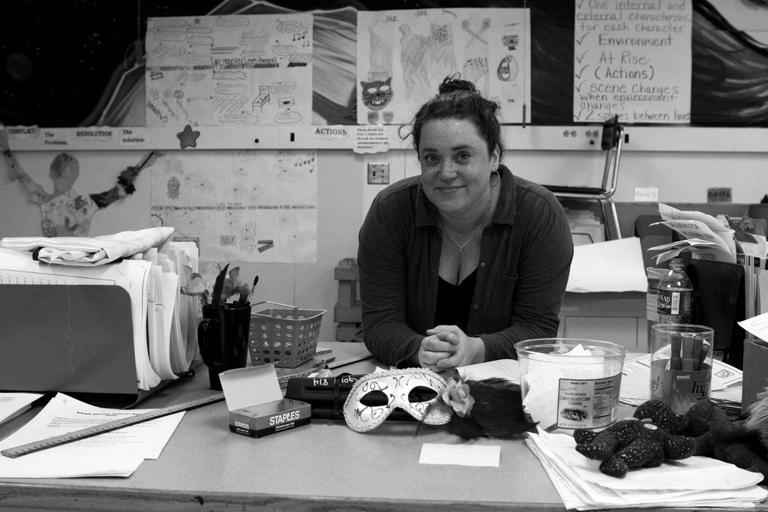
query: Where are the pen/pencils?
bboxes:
[312,356,336,368]
[212,263,259,310]
[316,349,333,355]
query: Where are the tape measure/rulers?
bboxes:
[0,352,376,459]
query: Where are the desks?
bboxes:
[0,340,765,511]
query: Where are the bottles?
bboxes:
[654,257,694,327]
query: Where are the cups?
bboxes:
[196,304,252,391]
[647,321,713,408]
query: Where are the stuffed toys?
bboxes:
[573,399,700,478]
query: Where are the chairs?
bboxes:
[537,116,624,244]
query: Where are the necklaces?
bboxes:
[438,202,494,255]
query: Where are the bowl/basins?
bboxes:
[511,338,626,438]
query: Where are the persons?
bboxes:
[354,76,574,375]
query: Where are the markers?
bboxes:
[670,333,709,373]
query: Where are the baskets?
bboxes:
[248,301,328,369]
